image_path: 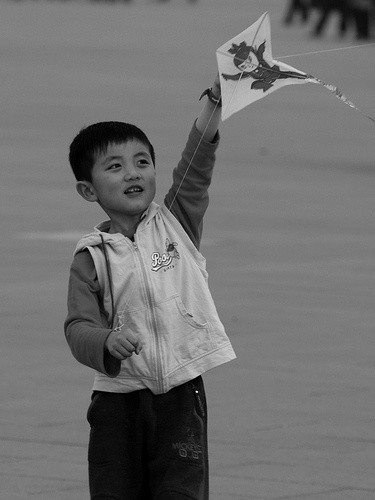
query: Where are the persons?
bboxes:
[64,75,236,499]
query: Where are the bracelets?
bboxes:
[199,87,222,108]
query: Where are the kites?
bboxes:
[216,10,360,124]
